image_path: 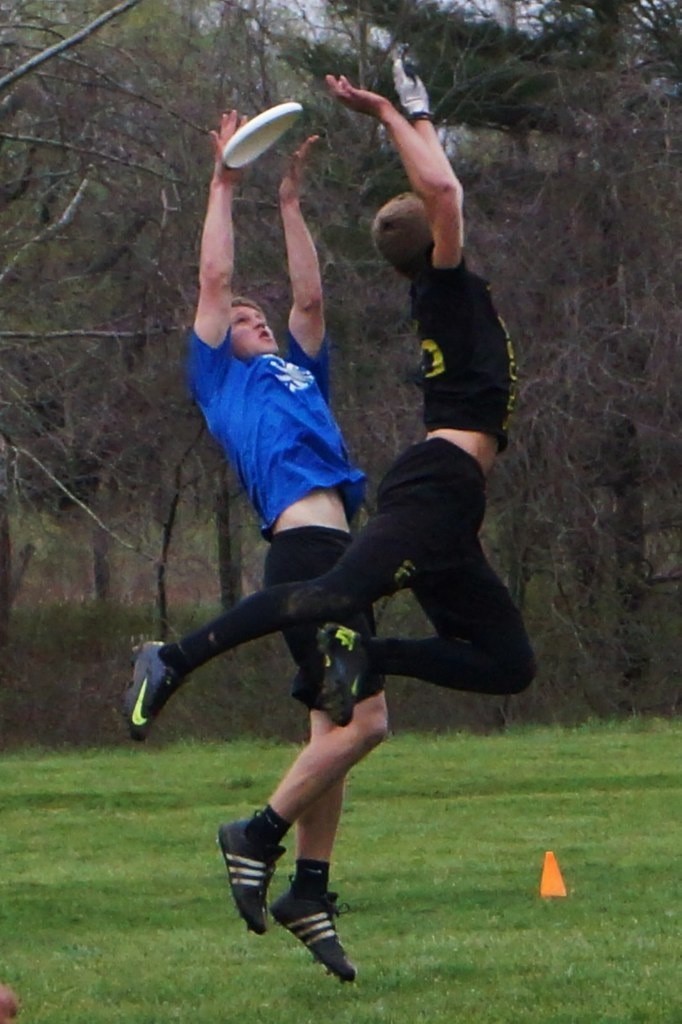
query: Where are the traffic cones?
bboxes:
[540,850,566,897]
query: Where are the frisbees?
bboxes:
[223,101,304,166]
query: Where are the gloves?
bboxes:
[391,53,431,117]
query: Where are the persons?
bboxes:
[185,109,389,982]
[125,42,535,747]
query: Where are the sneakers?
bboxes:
[217,818,285,937]
[271,866,358,985]
[318,620,368,725]
[125,642,182,740]
